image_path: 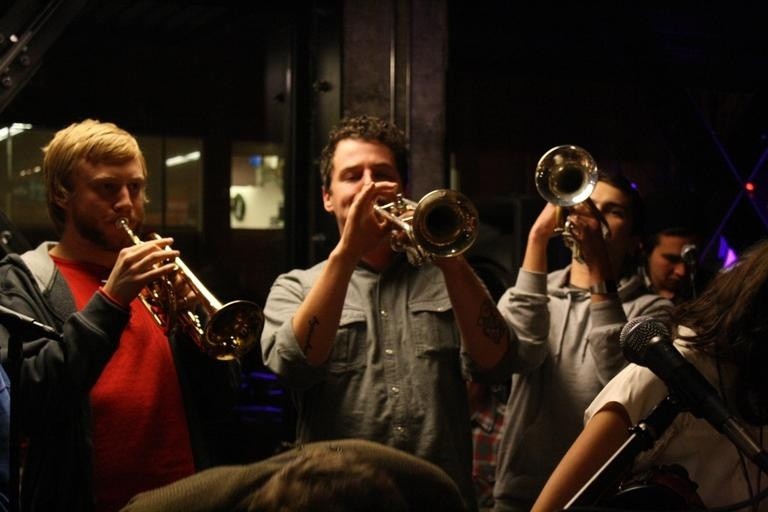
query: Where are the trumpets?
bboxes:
[115,217,264,361]
[536,146,610,263]
[373,189,480,266]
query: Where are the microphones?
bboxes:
[618,312,768,465]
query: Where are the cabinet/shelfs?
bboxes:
[251,0,341,299]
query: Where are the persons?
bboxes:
[258,114,509,512]
[0,118,296,511]
[643,225,696,304]
[528,236,768,512]
[496,169,672,511]
